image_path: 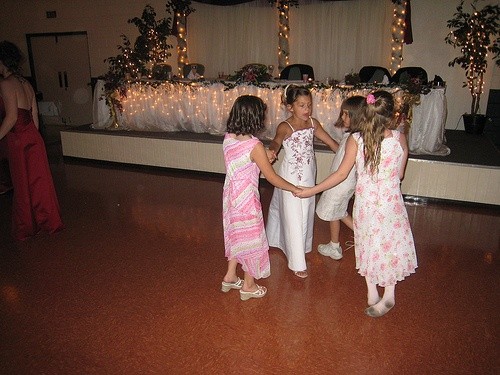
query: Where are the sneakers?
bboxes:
[317,240,343,260]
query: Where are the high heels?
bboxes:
[240,283,268,300]
[222,276,244,292]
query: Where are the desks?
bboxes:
[94,81,450,156]
[359,66,392,84]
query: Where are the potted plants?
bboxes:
[447,1,500,132]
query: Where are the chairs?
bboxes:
[392,66,429,83]
[151,64,172,79]
[183,64,204,78]
[279,63,315,80]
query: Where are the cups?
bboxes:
[137,72,141,79]
[125,73,130,81]
[325,78,330,85]
[168,72,172,80]
[303,74,308,83]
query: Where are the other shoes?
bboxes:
[294,270,308,278]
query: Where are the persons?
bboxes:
[221,94,303,300]
[315,95,367,260]
[0,39,65,241]
[265,83,340,278]
[295,91,419,318]
